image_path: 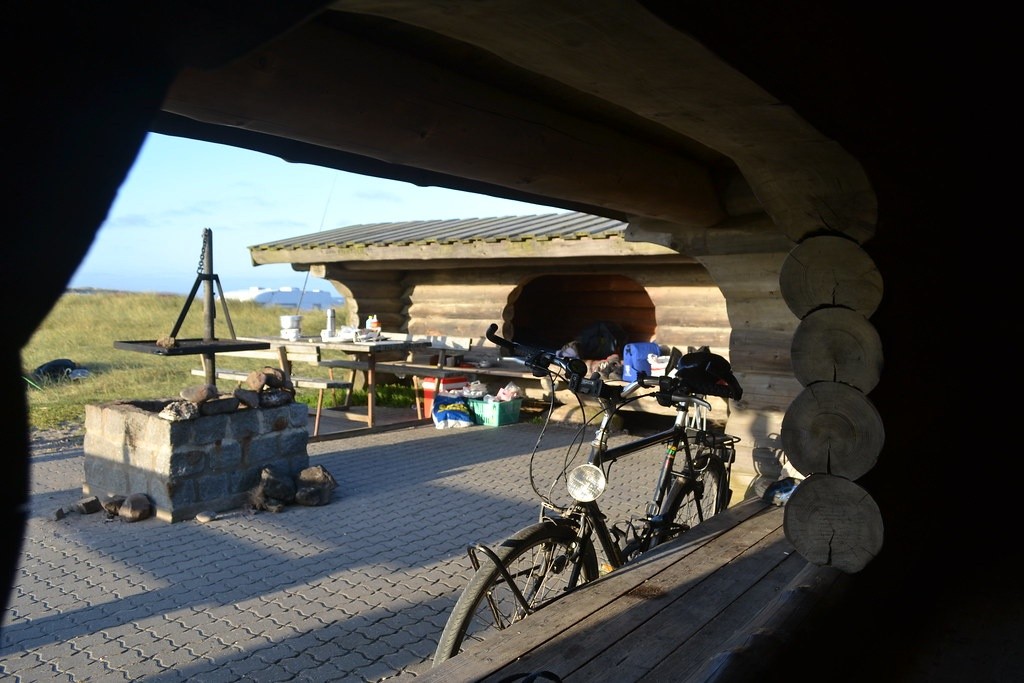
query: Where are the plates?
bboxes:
[327,338,344,343]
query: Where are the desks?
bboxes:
[236,335,432,427]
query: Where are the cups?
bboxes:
[321,330,327,343]
[288,329,299,342]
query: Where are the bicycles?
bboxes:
[433,323,742,666]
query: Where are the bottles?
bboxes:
[366,316,373,329]
[327,309,335,338]
[370,314,381,336]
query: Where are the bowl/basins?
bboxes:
[281,316,303,329]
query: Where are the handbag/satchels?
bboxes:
[647,353,670,377]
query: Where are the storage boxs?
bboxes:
[423,376,467,419]
[466,396,523,426]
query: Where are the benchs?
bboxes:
[310,327,472,420]
[188,335,354,436]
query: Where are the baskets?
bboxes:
[468,396,524,427]
